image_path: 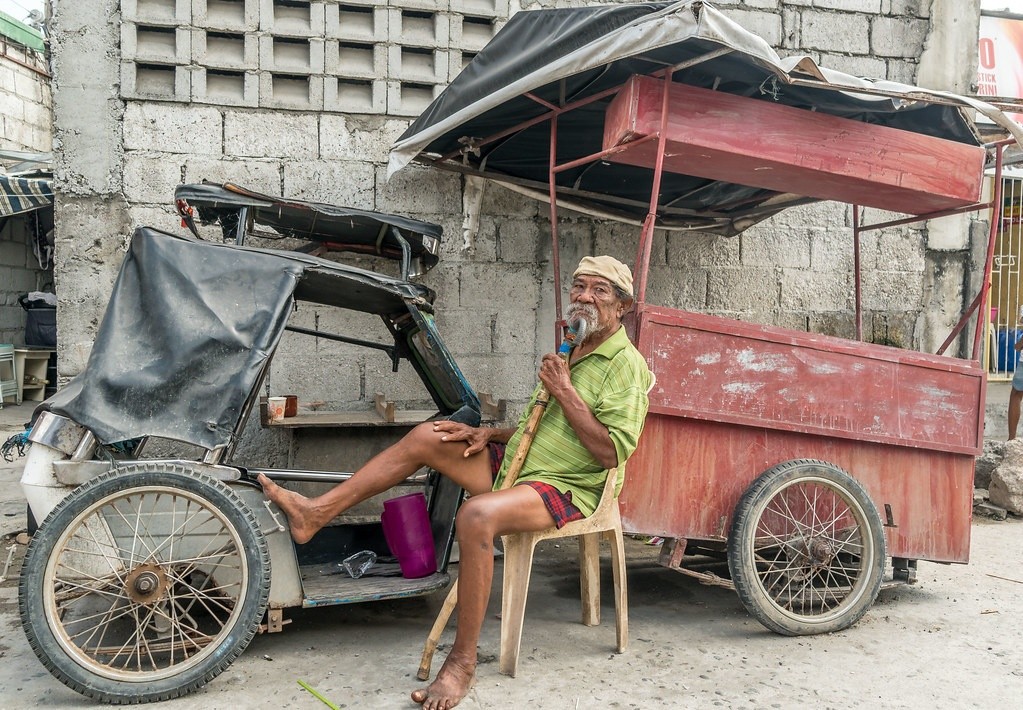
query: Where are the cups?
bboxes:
[278,395,297,417]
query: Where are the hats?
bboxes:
[573,253,636,297]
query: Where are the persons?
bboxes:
[1008,334,1023,440]
[258,255,651,710]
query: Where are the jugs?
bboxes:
[381,492,436,579]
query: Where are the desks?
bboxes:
[246,389,515,526]
[15,344,57,404]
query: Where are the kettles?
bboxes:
[268,396,287,421]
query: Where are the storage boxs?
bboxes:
[600,73,986,214]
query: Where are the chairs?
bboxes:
[418,468,631,684]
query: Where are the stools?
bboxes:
[0,344,19,409]
